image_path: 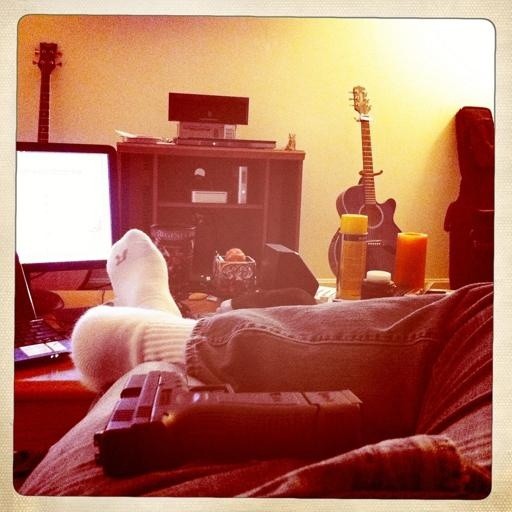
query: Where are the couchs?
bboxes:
[14,282,493,498]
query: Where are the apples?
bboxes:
[225,247,246,262]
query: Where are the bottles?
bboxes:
[361,270,396,300]
[336,214,368,300]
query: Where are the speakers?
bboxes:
[168,92,250,127]
[260,243,320,297]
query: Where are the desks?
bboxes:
[14,304,116,455]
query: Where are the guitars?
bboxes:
[328,86,402,280]
[29,41,94,291]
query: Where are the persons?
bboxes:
[18,229,493,499]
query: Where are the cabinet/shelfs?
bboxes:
[117,138,305,269]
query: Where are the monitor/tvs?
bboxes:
[15,141,118,316]
